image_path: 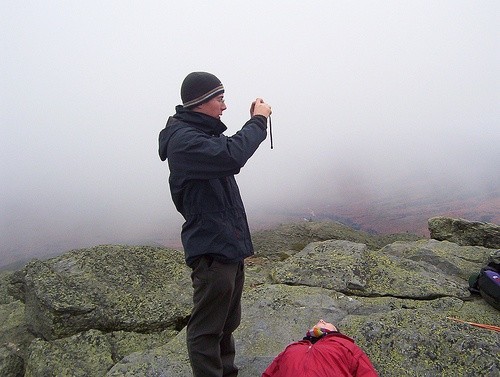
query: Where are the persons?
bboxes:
[259,319,378,377]
[158,72,272,377]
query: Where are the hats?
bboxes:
[181,72,224,108]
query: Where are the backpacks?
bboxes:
[468,248,500,312]
